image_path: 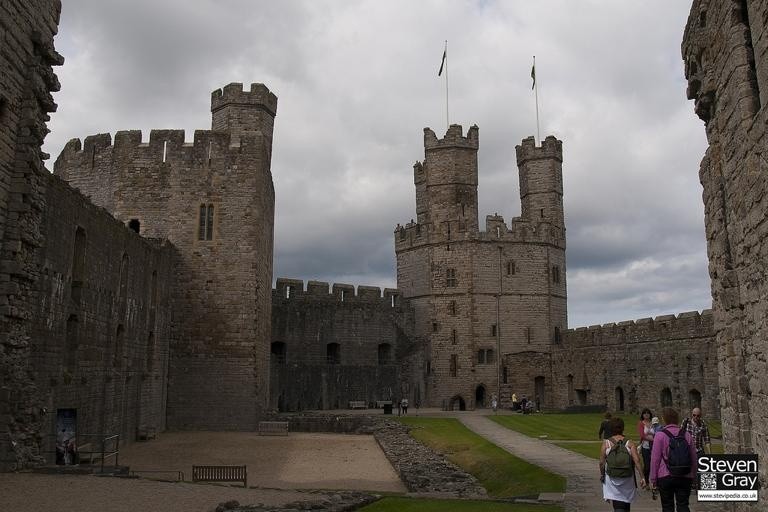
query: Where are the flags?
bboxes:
[531,63,535,87]
[437,48,447,77]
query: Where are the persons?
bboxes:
[490,392,497,412]
[396,396,421,416]
[598,406,713,512]
[512,392,540,415]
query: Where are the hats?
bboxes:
[605,413,612,417]
[652,417,659,424]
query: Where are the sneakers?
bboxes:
[646,483,650,490]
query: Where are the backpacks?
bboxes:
[607,436,634,478]
[661,428,692,476]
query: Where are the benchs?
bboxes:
[348,400,368,410]
[258,421,289,435]
[191,464,248,490]
[376,401,395,409]
[129,470,185,480]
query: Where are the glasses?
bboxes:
[692,414,700,417]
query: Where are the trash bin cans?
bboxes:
[384,404,392,414]
